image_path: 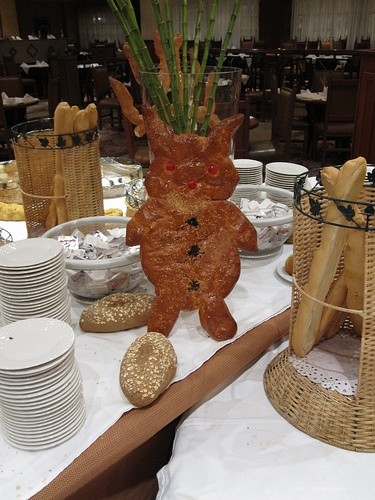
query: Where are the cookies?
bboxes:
[125,103,258,341]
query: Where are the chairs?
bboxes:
[0,34,375,164]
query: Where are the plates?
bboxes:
[302,176,324,191]
[232,159,262,206]
[0,237,71,325]
[0,318,87,452]
[265,162,308,209]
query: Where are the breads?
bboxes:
[119,331,177,408]
[289,157,367,357]
[79,292,156,332]
[44,102,99,227]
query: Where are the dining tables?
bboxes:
[1,154,375,500]
[22,64,99,99]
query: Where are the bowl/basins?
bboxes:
[39,216,145,299]
[100,157,144,198]
[229,184,294,258]
